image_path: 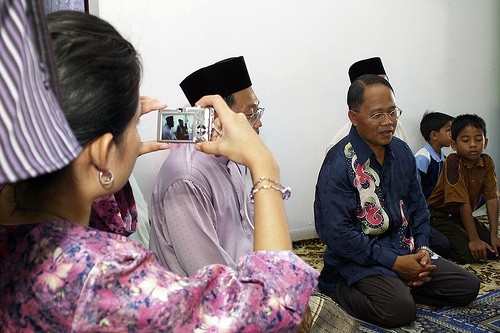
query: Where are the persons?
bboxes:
[0,10,320,333]
[149,56,359,333]
[413,112,453,199]
[314,57,481,329]
[176,118,189,140]
[162,116,176,140]
[427,114,500,263]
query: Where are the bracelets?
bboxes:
[250,177,290,201]
[417,246,434,256]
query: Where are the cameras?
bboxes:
[155,107,215,145]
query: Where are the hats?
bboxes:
[178,55,253,107]
[348,57,386,84]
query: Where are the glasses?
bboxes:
[353,106,403,123]
[246,107,265,126]
